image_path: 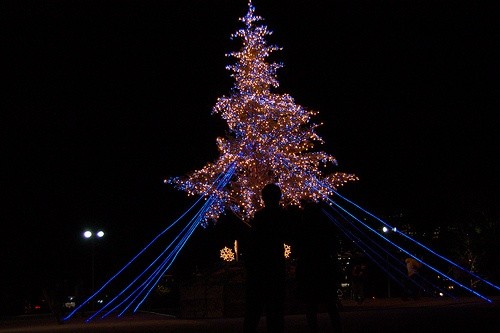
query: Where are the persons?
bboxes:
[401,252,444,302]
[241,184,342,333]
